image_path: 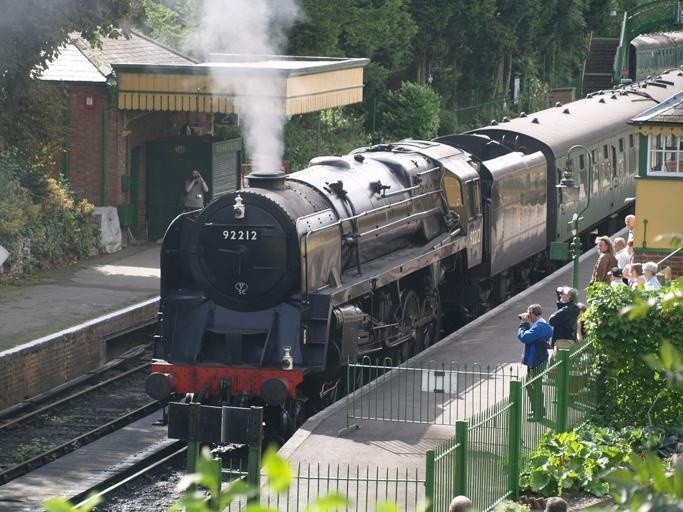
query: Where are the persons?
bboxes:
[548,285,579,402]
[182,165,209,213]
[573,213,660,345]
[515,303,553,422]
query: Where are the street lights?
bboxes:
[554,144,591,304]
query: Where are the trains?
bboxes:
[145,64,683,469]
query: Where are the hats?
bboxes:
[606,266,623,277]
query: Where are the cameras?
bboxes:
[517,313,529,320]
[193,175,201,180]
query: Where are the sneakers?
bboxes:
[526,407,546,422]
